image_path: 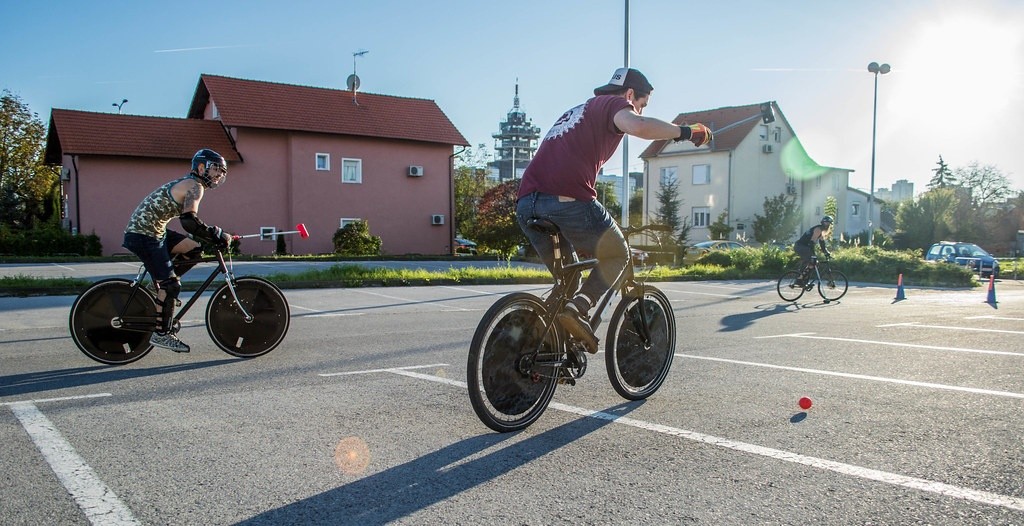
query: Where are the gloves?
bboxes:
[674,121,715,148]
[214,225,232,247]
[826,252,830,258]
[812,257,817,264]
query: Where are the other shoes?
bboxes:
[794,278,803,286]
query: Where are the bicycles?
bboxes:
[777,248,849,301]
[69,234,290,365]
[466,219,677,433]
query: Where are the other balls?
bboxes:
[799,397,812,409]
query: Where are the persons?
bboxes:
[513,69,713,387]
[122,147,234,352]
[791,216,834,289]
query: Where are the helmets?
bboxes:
[190,148,227,190]
[821,215,833,223]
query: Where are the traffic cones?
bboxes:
[893,274,908,299]
[984,275,998,303]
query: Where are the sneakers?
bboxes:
[149,332,190,353]
[557,299,599,354]
[145,281,181,306]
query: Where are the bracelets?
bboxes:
[673,125,691,142]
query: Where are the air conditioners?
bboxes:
[63,218,71,230]
[762,144,774,153]
[788,186,798,195]
[408,165,424,177]
[61,168,69,181]
[431,215,445,225]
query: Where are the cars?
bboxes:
[630,246,649,267]
[926,241,1000,278]
[456,229,477,257]
[683,240,746,267]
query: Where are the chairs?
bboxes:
[946,248,953,255]
[962,249,968,256]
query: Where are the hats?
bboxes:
[593,68,653,96]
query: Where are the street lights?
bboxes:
[867,62,891,246]
